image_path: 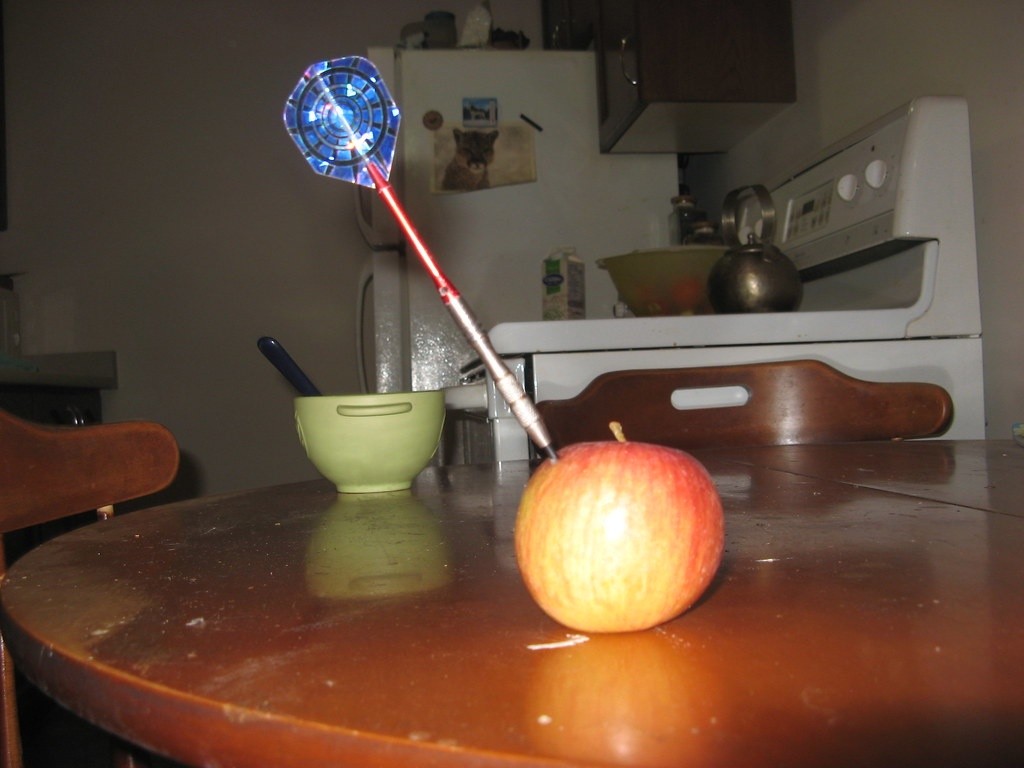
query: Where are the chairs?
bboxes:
[531,358,955,458]
[0,405,181,768]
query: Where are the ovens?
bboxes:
[441,337,986,465]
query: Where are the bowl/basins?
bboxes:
[293,390,445,494]
[595,244,730,316]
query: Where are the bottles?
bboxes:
[668,195,721,244]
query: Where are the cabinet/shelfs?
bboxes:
[539,0,799,152]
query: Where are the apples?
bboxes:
[513,420,725,635]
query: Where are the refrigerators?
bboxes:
[354,47,681,393]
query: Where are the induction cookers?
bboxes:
[459,94,980,372]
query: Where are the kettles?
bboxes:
[707,183,802,314]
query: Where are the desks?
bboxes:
[0,437,1024,768]
